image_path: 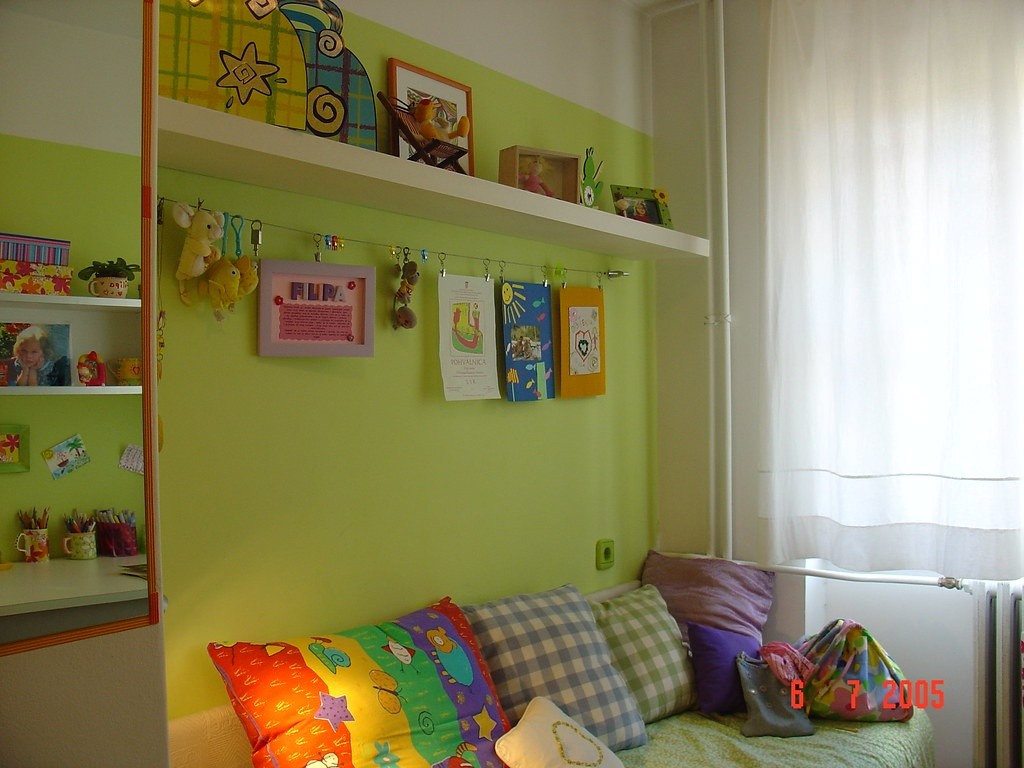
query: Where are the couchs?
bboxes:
[168,551,935,768]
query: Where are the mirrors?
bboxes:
[0,0,158,655]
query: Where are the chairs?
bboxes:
[378,92,469,176]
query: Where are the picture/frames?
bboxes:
[610,184,673,230]
[388,57,475,177]
[256,258,376,359]
[0,424,30,474]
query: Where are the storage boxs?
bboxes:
[0,259,74,296]
[498,144,581,205]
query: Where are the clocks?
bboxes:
[580,146,603,209]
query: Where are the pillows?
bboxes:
[494,698,625,768]
[689,623,763,713]
[204,596,512,768]
[458,581,647,754]
[641,549,775,647]
[586,584,698,724]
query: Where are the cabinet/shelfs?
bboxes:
[0,293,148,616]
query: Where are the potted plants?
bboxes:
[78,258,142,298]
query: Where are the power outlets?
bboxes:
[595,538,615,570]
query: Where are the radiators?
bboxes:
[939,577,1024,768]
